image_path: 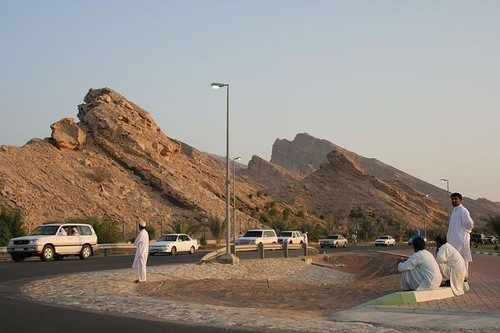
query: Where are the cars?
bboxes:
[149,234,198,255]
[408,236,428,245]
[471,233,497,244]
[320,235,348,248]
[375,236,395,247]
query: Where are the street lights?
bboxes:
[441,178,450,222]
[210,82,241,264]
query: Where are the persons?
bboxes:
[398,236,442,291]
[132,221,149,283]
[435,234,467,296]
[351,232,358,247]
[446,193,474,281]
[238,232,243,238]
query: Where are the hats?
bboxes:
[140,220,146,227]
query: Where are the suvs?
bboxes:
[238,230,305,247]
[7,223,98,262]
